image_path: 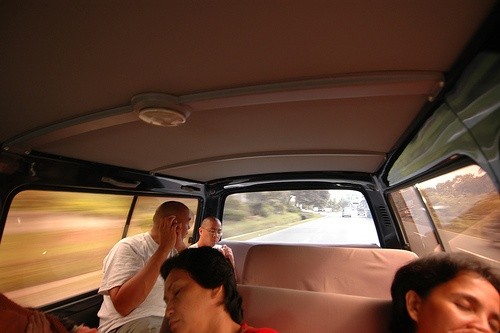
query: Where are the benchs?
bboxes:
[159,283,396,333]
[242,245,418,299]
[218,240,379,284]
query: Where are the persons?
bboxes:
[390,251,500,333]
[159,247,278,333]
[97,201,193,333]
[188,217,237,279]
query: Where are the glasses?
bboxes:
[202,227,223,235]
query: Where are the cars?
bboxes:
[342,207,352,218]
[358,209,365,215]
